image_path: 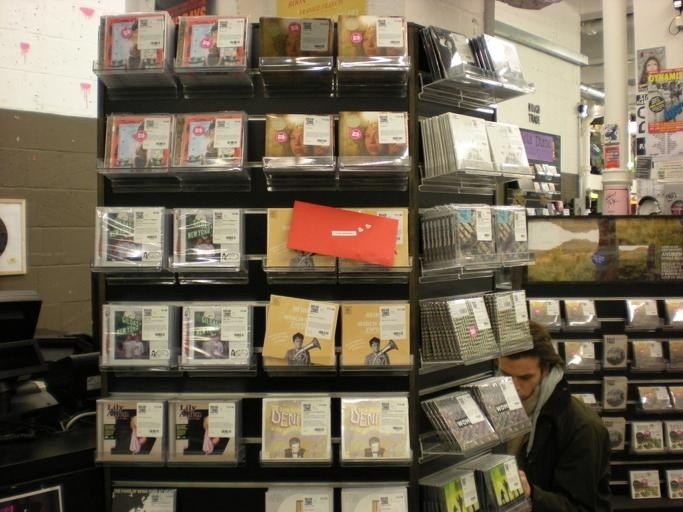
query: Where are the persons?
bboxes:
[364,337,390,366]
[284,437,306,458]
[285,333,310,367]
[639,57,660,84]
[498,320,611,512]
[286,32,305,56]
[364,437,385,457]
[364,123,384,155]
[202,335,224,359]
[290,125,308,156]
[122,334,144,359]
[363,26,382,56]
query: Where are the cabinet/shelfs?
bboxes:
[527,214,683,508]
[93,13,540,508]
